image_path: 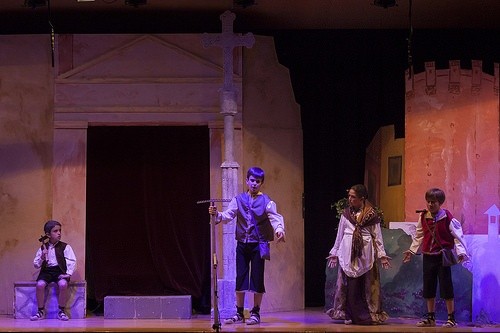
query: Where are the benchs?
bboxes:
[104,296,192,319]
[12,280,87,319]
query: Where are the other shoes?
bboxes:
[442,320,458,328]
[224,314,245,324]
[246,315,260,324]
[30,312,46,321]
[416,319,436,327]
[57,312,69,320]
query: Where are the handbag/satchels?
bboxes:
[441,248,458,265]
[259,242,270,260]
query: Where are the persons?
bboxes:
[30,220,77,320]
[402,189,469,328]
[209,167,286,325]
[325,184,392,324]
